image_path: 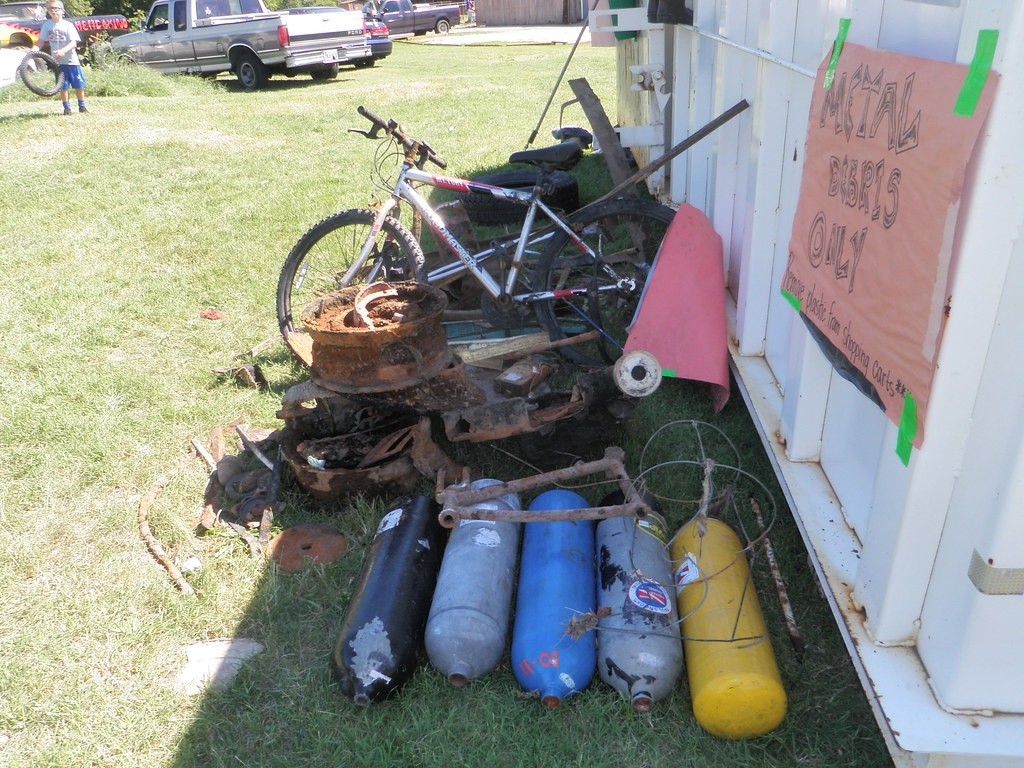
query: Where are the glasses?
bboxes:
[47,7,62,12]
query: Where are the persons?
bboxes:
[361,0,381,19]
[464,0,477,23]
[23,0,92,115]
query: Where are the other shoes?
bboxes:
[64,108,71,114]
[79,106,89,113]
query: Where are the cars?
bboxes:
[280,5,393,69]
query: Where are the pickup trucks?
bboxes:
[360,0,462,36]
[111,0,373,91]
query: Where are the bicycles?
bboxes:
[276,105,681,383]
